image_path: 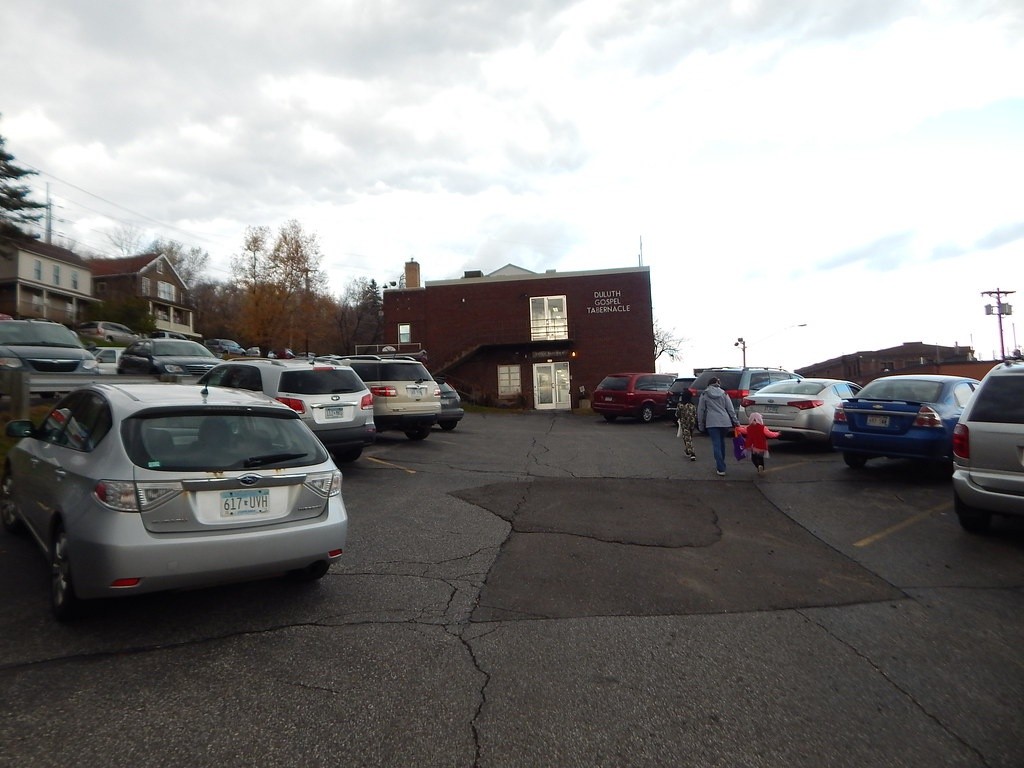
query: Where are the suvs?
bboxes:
[319,355,440,441]
[195,355,376,462]
[688,366,806,436]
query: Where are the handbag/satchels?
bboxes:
[676,419,682,437]
[733,434,746,461]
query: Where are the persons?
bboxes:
[675,389,697,461]
[697,377,741,476]
[734,412,782,478]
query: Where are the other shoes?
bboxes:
[758,465,765,478]
[715,463,727,475]
[683,450,691,457]
[691,454,696,461]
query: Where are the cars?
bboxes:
[737,378,864,453]
[87,346,124,377]
[590,373,678,424]
[78,320,139,343]
[117,338,227,384]
[0,369,349,620]
[665,378,697,428]
[0,319,100,399]
[950,356,1024,531]
[434,377,464,431]
[831,374,981,468]
[203,338,316,359]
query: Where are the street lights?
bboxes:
[981,287,1016,361]
[734,338,746,368]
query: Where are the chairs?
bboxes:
[185,417,255,467]
[139,428,185,468]
